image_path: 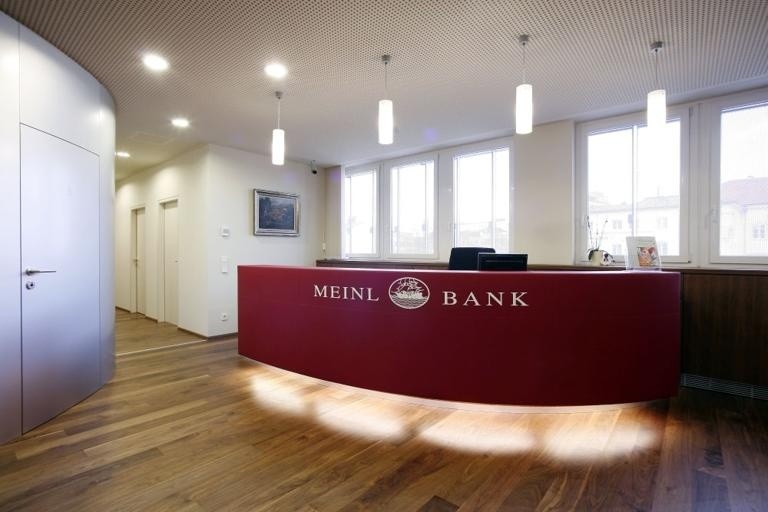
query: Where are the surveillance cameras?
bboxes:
[311,160,317,174]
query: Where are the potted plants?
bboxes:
[582,215,612,264]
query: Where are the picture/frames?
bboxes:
[248,187,301,239]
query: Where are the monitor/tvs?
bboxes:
[477,253,528,271]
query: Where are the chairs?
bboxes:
[447,246,495,270]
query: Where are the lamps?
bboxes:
[514,34,534,137]
[647,40,667,137]
[268,91,286,167]
[374,55,396,145]
